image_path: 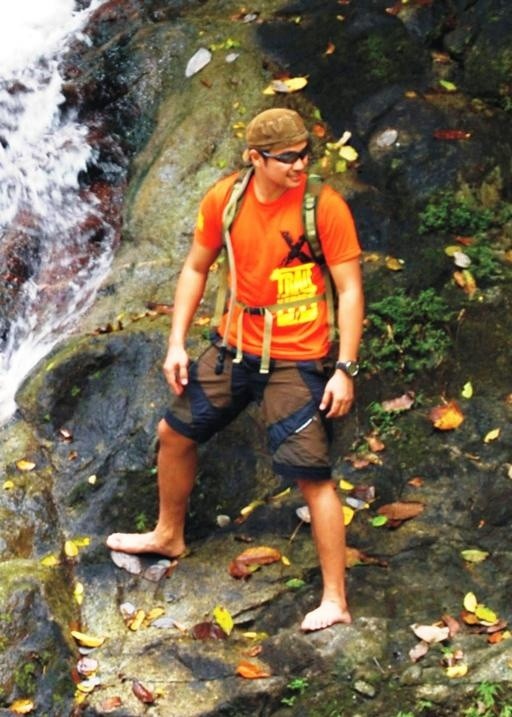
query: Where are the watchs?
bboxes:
[336,360,359,378]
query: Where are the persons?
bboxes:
[106,108,365,631]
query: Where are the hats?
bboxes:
[240,108,311,163]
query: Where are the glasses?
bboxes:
[260,143,312,164]
